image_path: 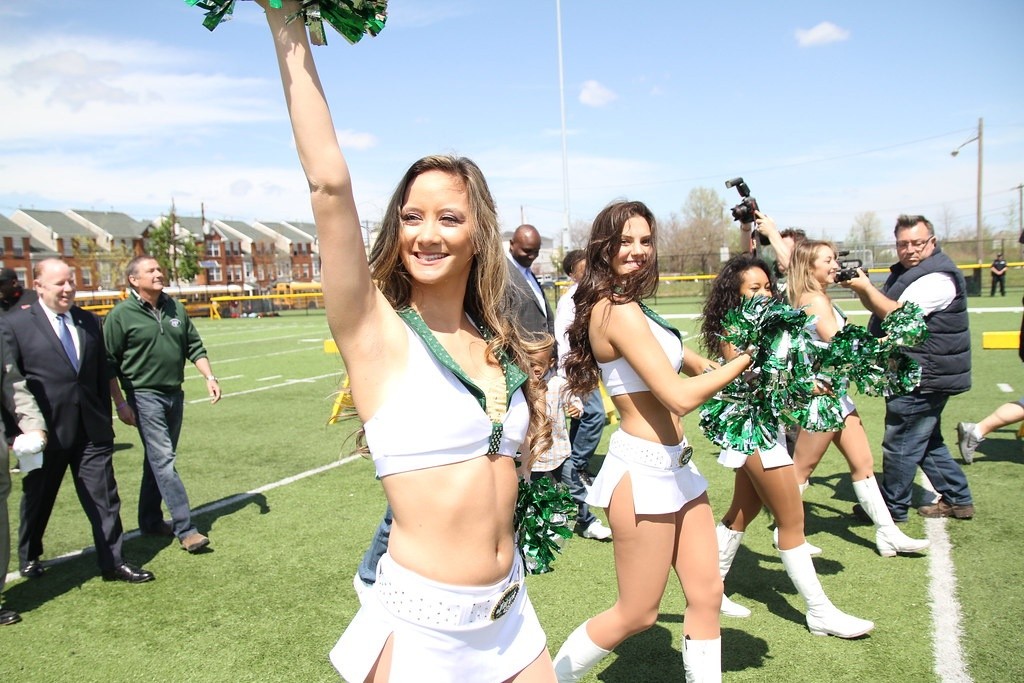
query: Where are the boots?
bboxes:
[852,475,930,558]
[553,619,614,683]
[716,522,752,619]
[773,479,822,555]
[681,634,722,683]
[778,540,874,638]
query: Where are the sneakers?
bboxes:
[958,422,986,465]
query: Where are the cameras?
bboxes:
[725,177,760,223]
[833,251,869,283]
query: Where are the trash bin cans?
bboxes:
[963,275,981,296]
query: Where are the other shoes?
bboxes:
[583,519,612,539]
[352,572,371,605]
[579,472,594,493]
[140,522,175,538]
[853,504,907,522]
[180,532,209,552]
[919,496,974,518]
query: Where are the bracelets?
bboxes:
[207,376,219,383]
[116,403,126,410]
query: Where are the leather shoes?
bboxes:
[102,562,154,583]
[19,557,44,577]
[0,610,21,625]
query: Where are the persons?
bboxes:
[954,294,1024,465]
[773,241,931,558]
[554,249,612,540]
[0,337,47,626]
[551,200,761,683]
[739,210,807,288]
[989,252,1007,297]
[261,0,558,683]
[0,258,156,583]
[841,214,976,521]
[697,254,875,639]
[103,255,221,551]
[527,351,584,539]
[353,499,394,601]
[0,268,38,335]
[501,224,554,343]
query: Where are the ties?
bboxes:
[526,269,547,317]
[55,314,79,373]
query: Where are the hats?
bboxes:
[0,268,17,280]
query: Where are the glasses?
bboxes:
[894,235,933,251]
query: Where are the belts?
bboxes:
[374,560,524,631]
[608,433,694,468]
